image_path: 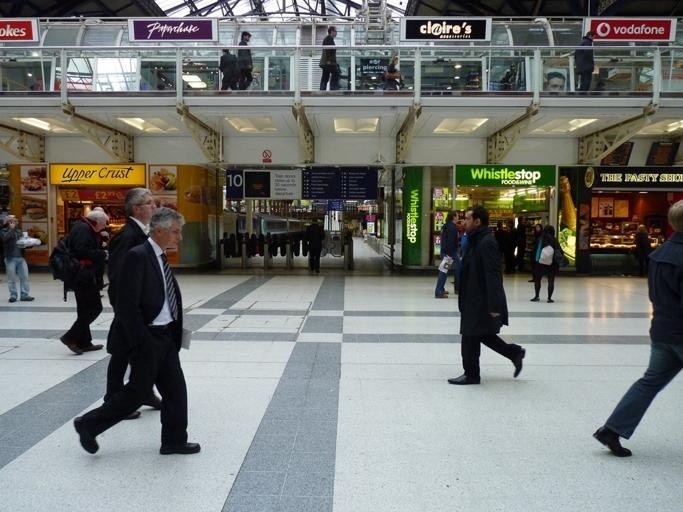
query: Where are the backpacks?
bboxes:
[48,235,80,279]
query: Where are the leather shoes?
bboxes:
[83,344,103,351]
[160,443,200,454]
[144,394,162,408]
[59,335,82,355]
[593,427,632,457]
[448,372,480,384]
[126,411,139,420]
[74,416,99,454]
[513,347,525,378]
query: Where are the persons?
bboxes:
[0,217,35,302]
[383,57,399,91]
[504,66,516,90]
[102,188,161,419]
[435,210,459,298]
[547,72,565,92]
[528,223,543,282]
[504,221,520,274]
[237,31,253,90]
[460,229,468,251]
[593,197,683,456]
[218,49,237,90]
[74,207,200,454]
[93,207,109,298]
[574,31,595,96]
[319,26,339,90]
[59,211,107,355]
[529,225,564,303]
[447,205,526,385]
[8,215,16,219]
[306,218,324,273]
[633,224,651,277]
[517,216,532,271]
[494,221,508,254]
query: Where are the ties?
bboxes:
[161,253,178,321]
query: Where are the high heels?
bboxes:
[547,299,554,302]
[531,297,540,302]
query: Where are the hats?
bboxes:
[3,214,18,225]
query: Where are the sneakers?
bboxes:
[444,291,448,294]
[9,297,17,302]
[441,294,448,298]
[20,295,34,300]
